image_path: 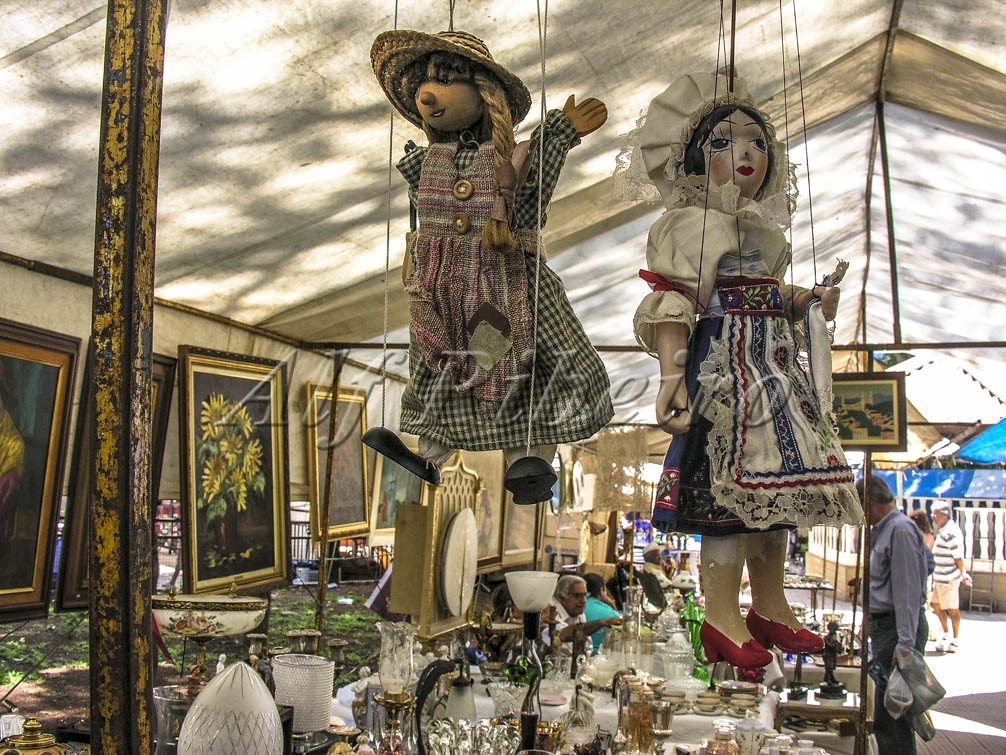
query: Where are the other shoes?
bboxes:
[934,638,959,653]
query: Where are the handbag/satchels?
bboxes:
[883,664,913,719]
[894,641,947,718]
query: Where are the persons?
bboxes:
[611,69,868,673]
[852,471,929,755]
[642,542,678,588]
[490,573,624,655]
[909,503,971,652]
[360,29,616,505]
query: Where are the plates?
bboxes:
[695,709,722,716]
[673,707,687,714]
[729,711,753,717]
[540,700,566,705]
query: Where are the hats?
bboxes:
[642,542,659,555]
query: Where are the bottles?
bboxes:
[705,720,739,755]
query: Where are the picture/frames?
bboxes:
[832,372,907,452]
[178,344,294,594]
[0,317,82,625]
[54,336,177,613]
[307,382,371,542]
[462,449,547,575]
[370,435,425,536]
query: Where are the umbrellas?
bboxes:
[952,417,1006,465]
[872,355,1006,464]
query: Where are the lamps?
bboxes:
[504,571,559,751]
[150,594,268,679]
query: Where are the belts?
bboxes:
[867,612,894,620]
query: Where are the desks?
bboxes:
[776,688,860,734]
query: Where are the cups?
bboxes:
[151,685,203,755]
[799,750,813,755]
[798,740,813,748]
[487,682,529,722]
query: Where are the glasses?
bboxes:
[569,592,587,601]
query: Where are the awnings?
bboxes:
[857,467,1006,501]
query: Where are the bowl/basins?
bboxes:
[480,662,506,681]
[661,692,755,714]
